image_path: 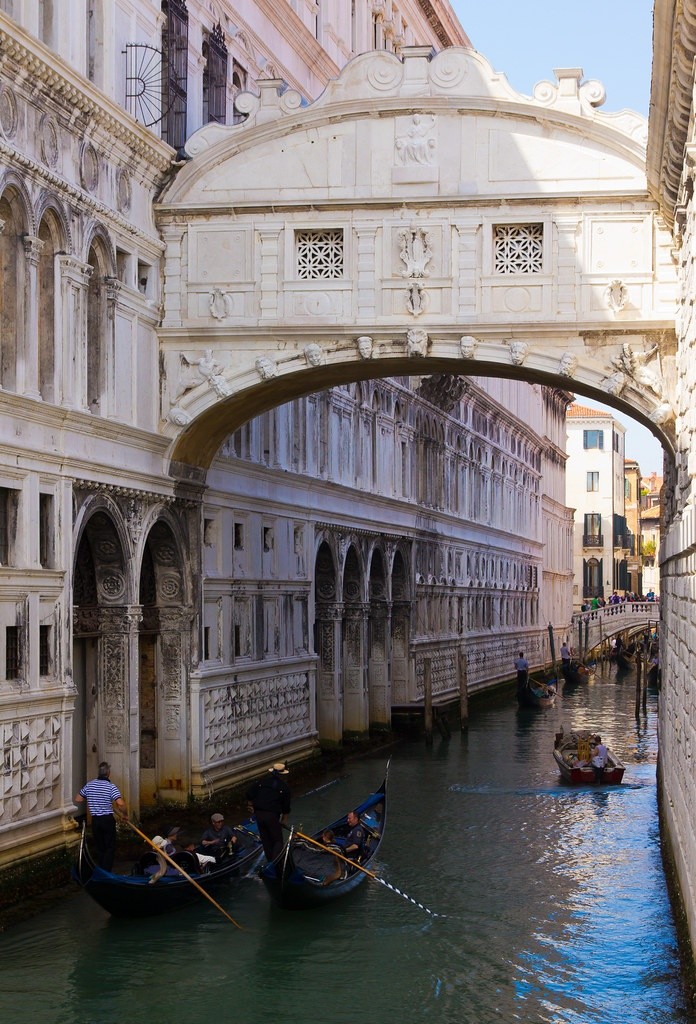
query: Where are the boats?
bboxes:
[559,660,597,683]
[615,642,637,669]
[70,816,263,916]
[515,678,566,708]
[638,636,659,653]
[553,725,626,786]
[258,758,392,903]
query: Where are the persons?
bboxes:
[643,630,656,644]
[615,634,623,656]
[581,591,660,621]
[245,763,291,874]
[74,762,130,873]
[514,652,528,688]
[561,643,571,664]
[139,813,238,878]
[342,810,365,859]
[318,829,348,857]
[553,736,608,787]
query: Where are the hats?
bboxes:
[97,761,112,775]
[211,813,225,822]
[151,835,168,849]
[165,826,180,836]
[268,763,290,776]
